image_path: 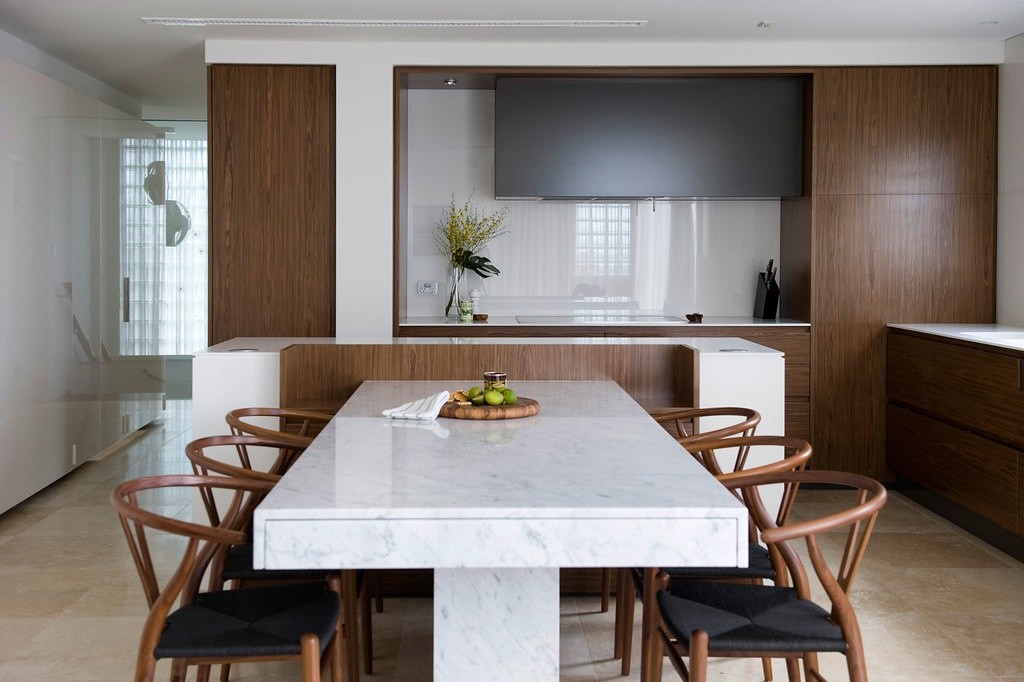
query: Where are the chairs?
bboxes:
[110,405,386,680]
[600,406,887,682]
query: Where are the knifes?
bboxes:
[765,259,777,290]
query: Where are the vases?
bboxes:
[450,262,476,322]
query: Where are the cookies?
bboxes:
[446,389,472,405]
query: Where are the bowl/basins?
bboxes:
[686,314,703,323]
[473,315,488,321]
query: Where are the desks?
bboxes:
[252,378,748,682]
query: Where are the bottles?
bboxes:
[459,300,473,321]
[469,288,480,314]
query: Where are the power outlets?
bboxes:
[417,280,438,295]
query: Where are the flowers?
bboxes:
[432,187,510,278]
